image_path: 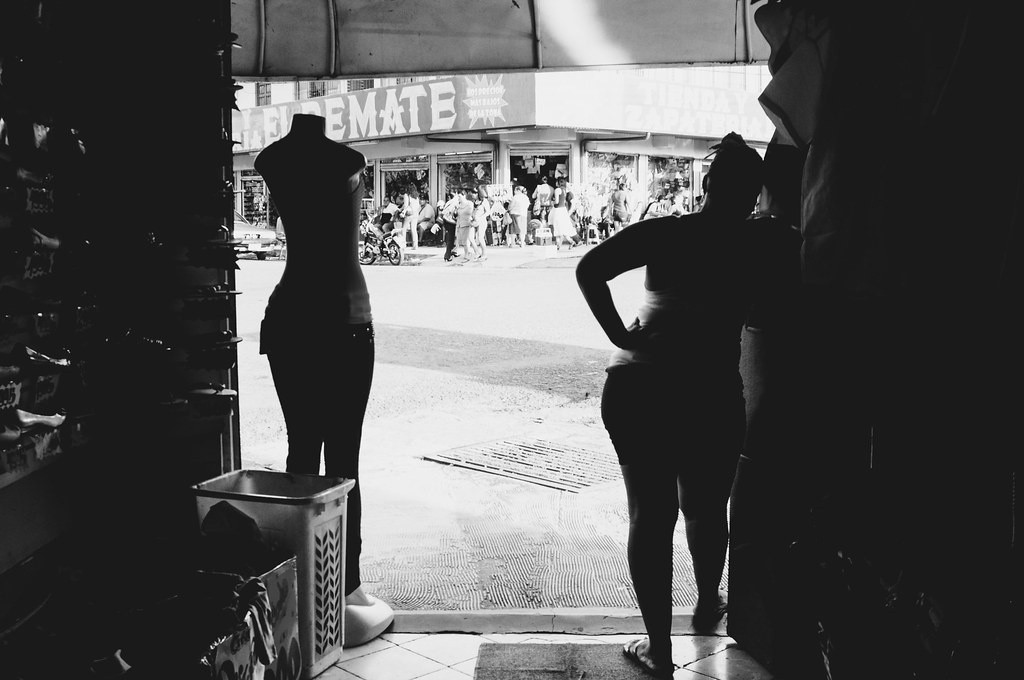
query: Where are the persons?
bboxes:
[571,133,798,677]
[249,112,391,631]
[362,168,700,265]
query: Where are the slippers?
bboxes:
[622,636,673,677]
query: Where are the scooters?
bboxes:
[357,218,405,266]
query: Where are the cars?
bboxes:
[233,207,276,263]
